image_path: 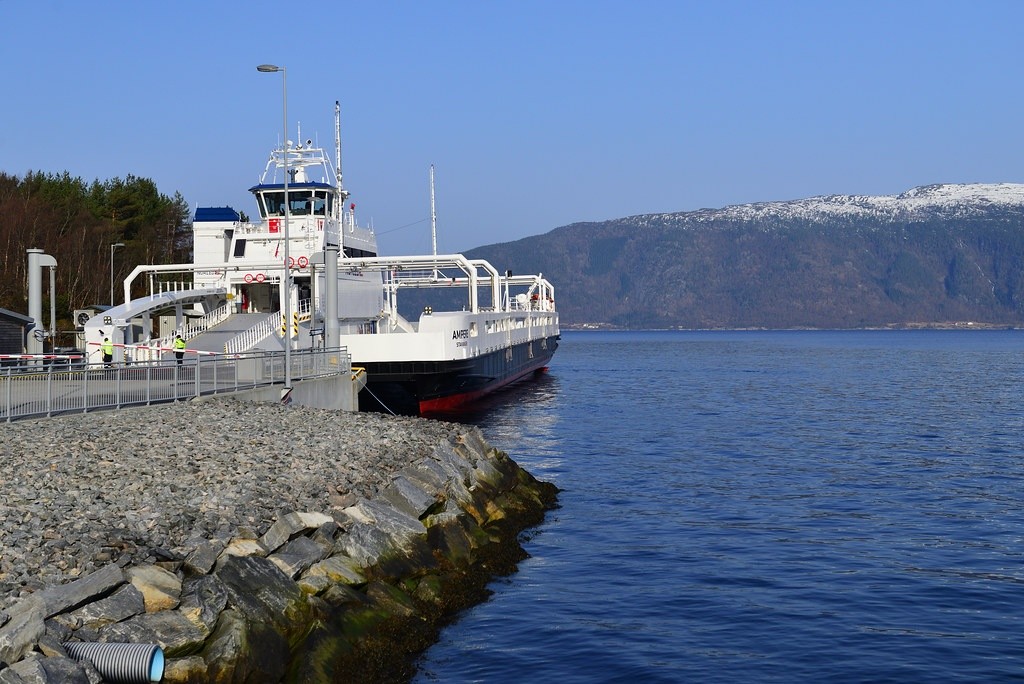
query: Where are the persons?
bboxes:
[101,338,113,373]
[172,329,174,335]
[242,290,248,313]
[280,208,285,215]
[173,335,185,370]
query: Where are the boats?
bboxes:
[79,96,563,417]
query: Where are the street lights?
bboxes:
[111,243,126,307]
[256,65,293,406]
